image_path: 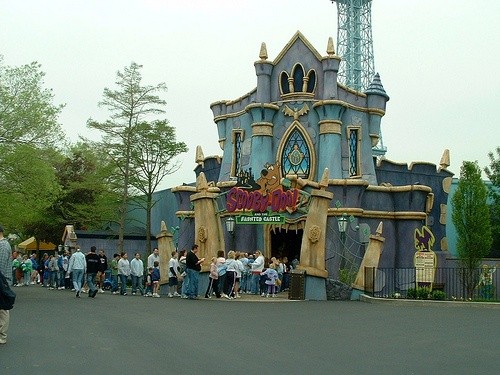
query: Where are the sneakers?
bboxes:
[168,293,173,297]
[173,291,180,296]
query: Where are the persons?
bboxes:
[12,245,300,299]
[0,227,13,344]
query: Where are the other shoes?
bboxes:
[72,287,105,294]
[88,290,97,297]
[192,295,200,299]
[13,281,34,286]
[181,294,189,299]
[76,290,81,298]
[208,289,277,300]
[40,283,65,290]
[112,291,160,298]
